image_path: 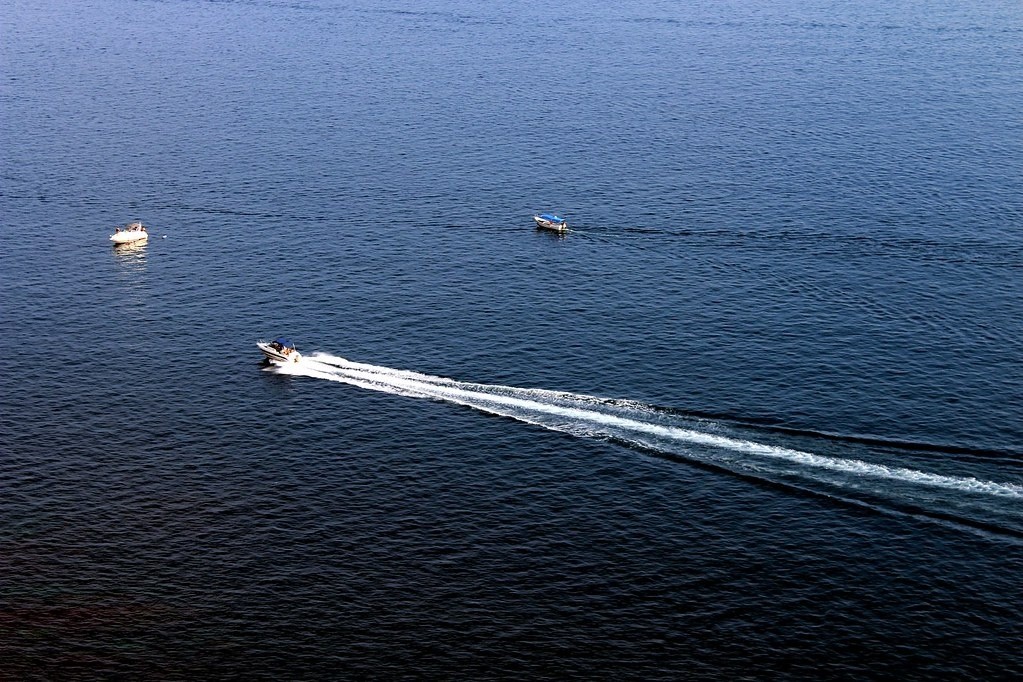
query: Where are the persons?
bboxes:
[116,226,146,236]
[550,220,567,229]
[270,341,294,356]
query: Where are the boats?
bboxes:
[256,338,301,365]
[534,214,566,231]
[110,230,148,243]
